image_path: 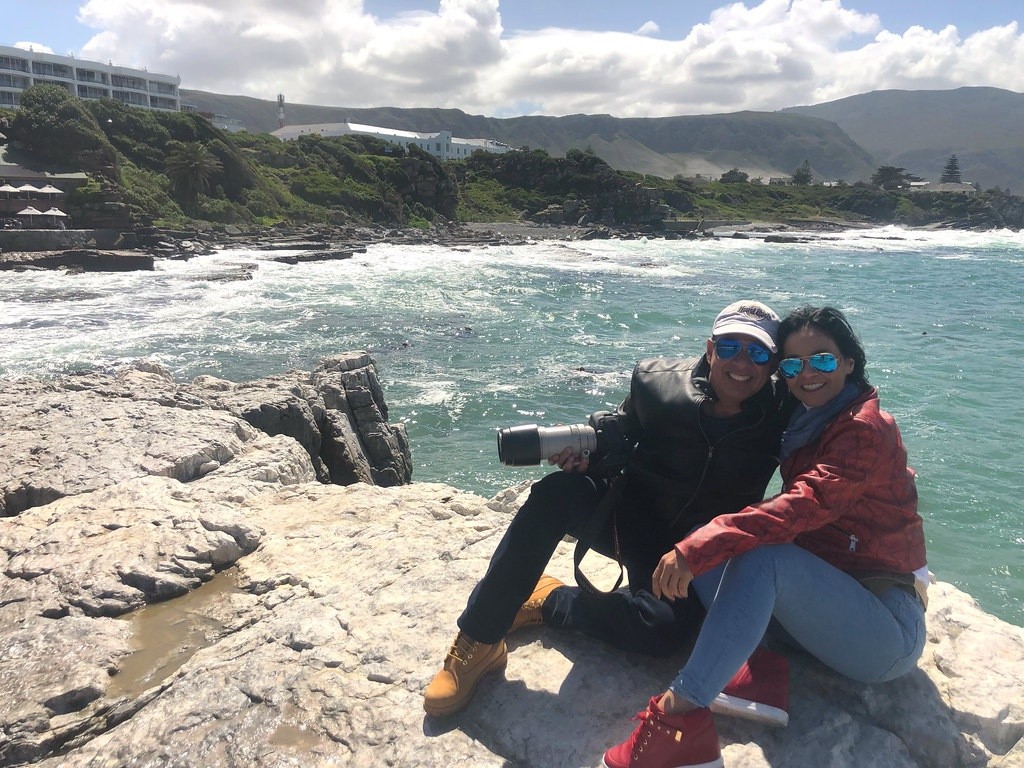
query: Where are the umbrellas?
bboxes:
[17,183,39,199]
[34,185,64,200]
[16,206,43,225]
[43,208,68,223]
[0,184,20,199]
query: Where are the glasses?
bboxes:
[711,335,773,366]
[779,352,845,380]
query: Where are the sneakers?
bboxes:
[502,574,567,634]
[708,645,791,728]
[422,629,507,717]
[602,692,723,768]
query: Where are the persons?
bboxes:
[55,215,73,230]
[603,302,928,768]
[423,301,808,718]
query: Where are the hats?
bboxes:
[712,300,785,354]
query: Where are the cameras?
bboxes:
[497,410,630,474]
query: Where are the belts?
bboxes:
[864,578,926,611]
